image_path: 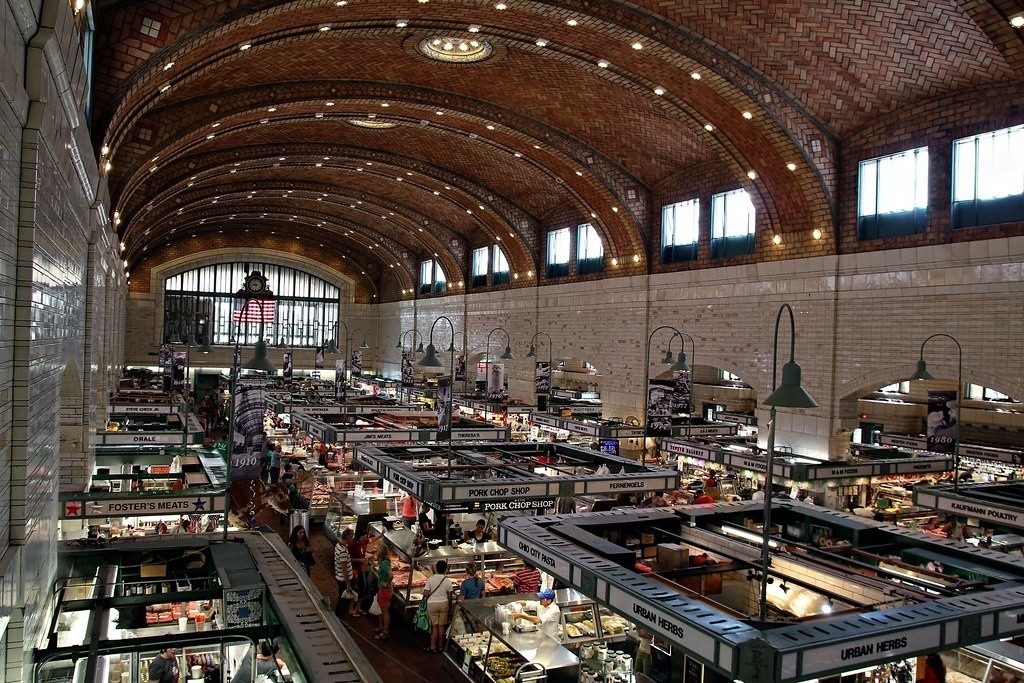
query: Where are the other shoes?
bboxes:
[356,609,366,615]
[349,611,360,617]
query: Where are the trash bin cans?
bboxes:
[289,509,310,539]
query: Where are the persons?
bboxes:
[550,435,560,442]
[148,648,179,683]
[372,545,394,640]
[458,563,485,633]
[231,640,283,683]
[188,393,224,433]
[424,560,453,654]
[942,516,970,542]
[705,469,717,487]
[515,559,542,593]
[169,519,190,534]
[400,492,434,539]
[751,484,773,500]
[465,518,491,561]
[200,600,217,625]
[258,412,328,510]
[512,588,561,645]
[633,629,653,675]
[287,525,316,578]
[695,490,714,504]
[334,527,386,618]
[959,468,975,482]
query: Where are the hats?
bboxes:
[281,473,293,482]
[536,589,555,601]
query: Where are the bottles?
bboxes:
[581,641,636,683]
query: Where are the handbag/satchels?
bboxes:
[369,595,383,615]
[413,597,432,634]
[341,590,358,601]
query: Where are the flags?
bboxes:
[190,515,201,528]
[208,515,220,528]
[233,298,275,323]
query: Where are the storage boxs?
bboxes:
[370,498,386,514]
[658,543,689,570]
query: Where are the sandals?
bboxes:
[374,632,391,640]
[372,627,383,633]
[423,647,438,654]
[436,646,444,653]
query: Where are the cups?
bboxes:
[191,665,202,678]
[502,622,510,634]
[178,617,188,631]
[121,672,129,683]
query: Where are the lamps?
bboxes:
[721,525,777,548]
[779,580,792,595]
[822,597,834,613]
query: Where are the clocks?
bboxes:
[247,275,265,295]
